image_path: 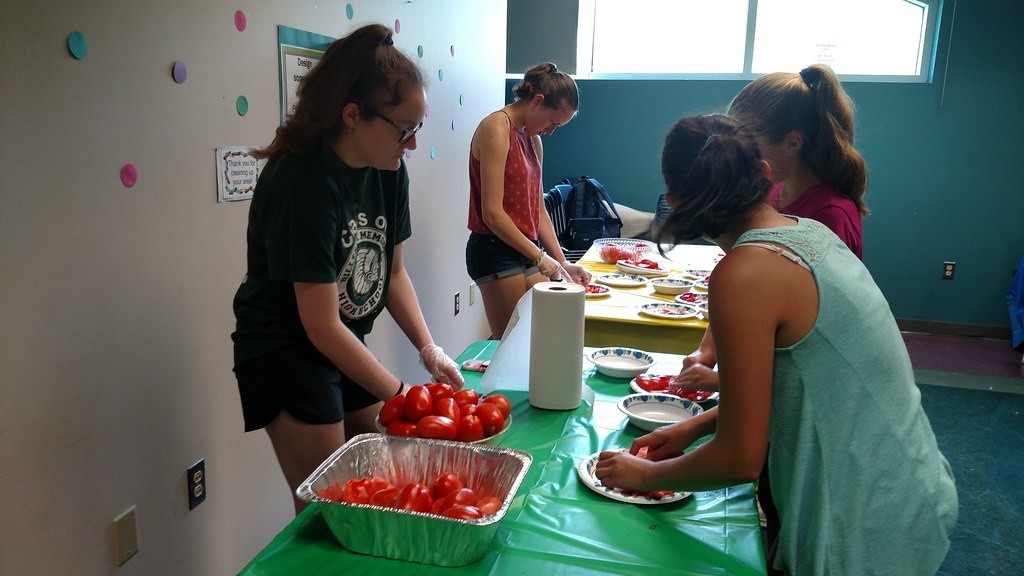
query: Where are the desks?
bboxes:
[575,243,727,356]
[225,340,770,576]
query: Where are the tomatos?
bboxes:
[584,285,606,293]
[614,447,675,498]
[601,242,659,270]
[337,471,499,521]
[378,382,510,442]
[635,374,712,400]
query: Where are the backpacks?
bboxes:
[561,176,623,249]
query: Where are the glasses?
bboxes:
[353,100,424,143]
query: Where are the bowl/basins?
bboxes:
[617,393,705,431]
[586,347,657,378]
[375,393,512,444]
[696,303,709,319]
[593,238,654,263]
[650,278,696,294]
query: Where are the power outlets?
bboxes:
[187,458,206,511]
[454,292,460,316]
[942,261,955,280]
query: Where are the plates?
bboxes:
[578,448,694,504]
[684,269,712,278]
[675,292,708,305]
[694,280,709,288]
[596,273,648,288]
[630,375,720,402]
[585,283,612,297]
[616,260,668,277]
[638,303,700,318]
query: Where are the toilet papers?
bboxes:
[481,280,586,411]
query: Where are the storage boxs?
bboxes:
[296,432,533,568]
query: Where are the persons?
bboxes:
[668,64,869,391]
[466,63,592,340]
[231,24,465,515]
[595,115,958,576]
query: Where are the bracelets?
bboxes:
[394,381,404,396]
